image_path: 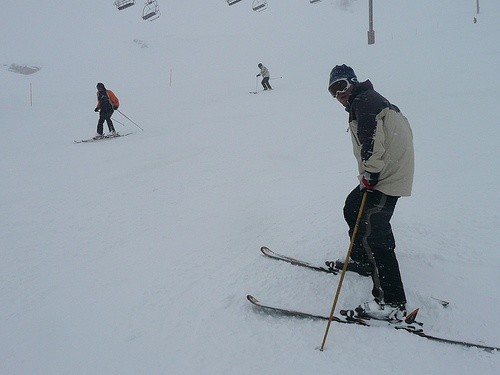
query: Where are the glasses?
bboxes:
[328,77,357,98]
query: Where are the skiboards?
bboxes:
[73,130,132,143]
[249,86,272,94]
[245,246,500,353]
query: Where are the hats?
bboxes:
[329,64,359,85]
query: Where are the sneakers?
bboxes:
[329,254,378,277]
[355,298,407,321]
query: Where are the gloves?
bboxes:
[94,108,98,112]
[113,106,117,110]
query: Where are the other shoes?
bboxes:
[92,134,103,140]
[107,131,116,137]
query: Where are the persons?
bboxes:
[328,65,414,318]
[256,63,273,91]
[94,83,119,140]
